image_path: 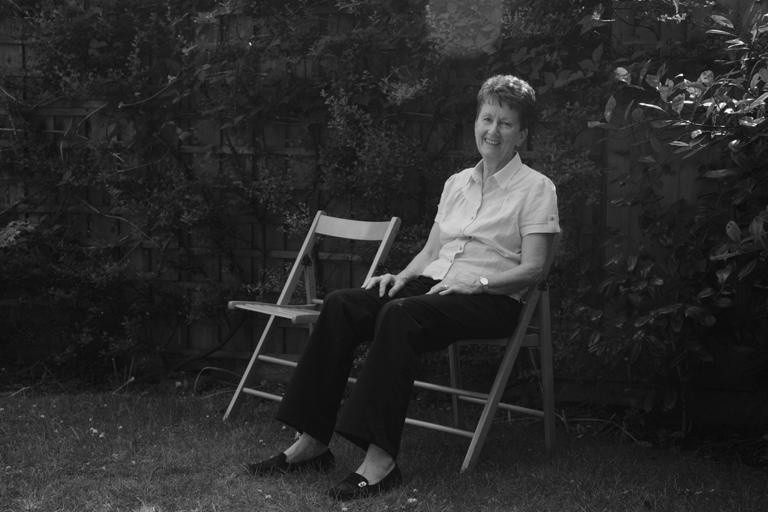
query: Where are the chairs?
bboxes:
[223,208,401,419]
[403,225,562,472]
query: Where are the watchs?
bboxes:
[480,275,490,293]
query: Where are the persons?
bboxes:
[245,73,562,500]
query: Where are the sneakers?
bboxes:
[328,462,404,498]
[248,447,336,476]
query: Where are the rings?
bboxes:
[444,285,449,290]
